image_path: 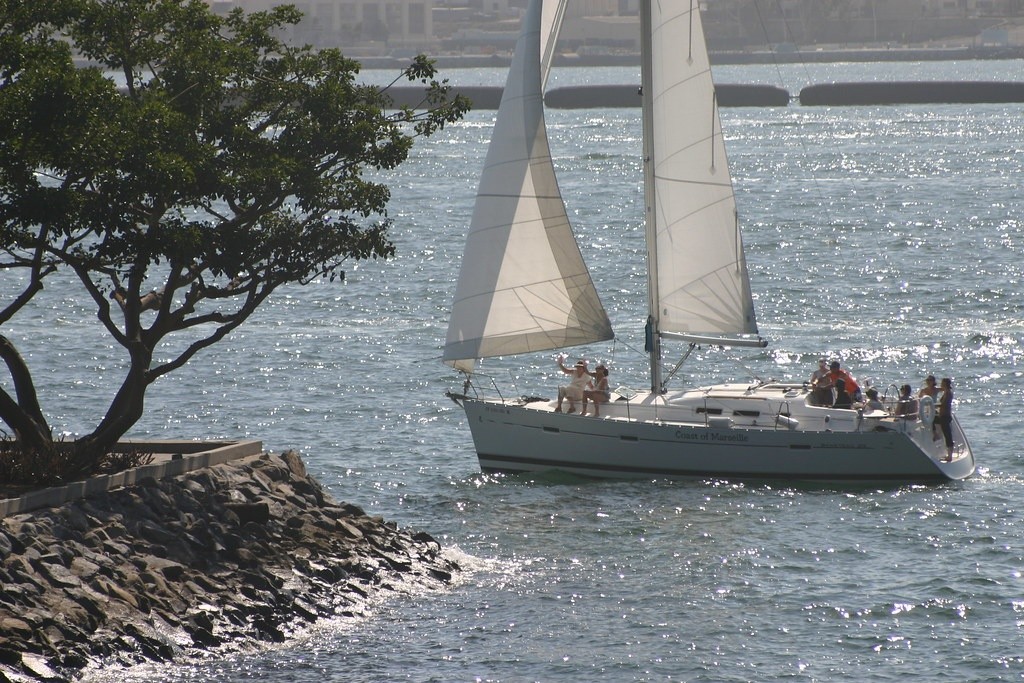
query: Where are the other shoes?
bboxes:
[581,411,586,415]
[568,407,576,413]
[555,407,562,412]
[944,457,953,461]
[594,413,600,418]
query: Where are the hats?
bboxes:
[827,361,840,368]
[866,389,878,398]
[574,361,587,367]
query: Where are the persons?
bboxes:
[896,385,918,420]
[864,388,887,412]
[919,376,942,403]
[581,360,611,418]
[811,358,863,408]
[832,378,851,409]
[555,355,593,414]
[933,378,954,461]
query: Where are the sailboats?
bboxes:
[443,0,975,481]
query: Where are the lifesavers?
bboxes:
[920,395,935,424]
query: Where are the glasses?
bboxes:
[926,379,933,381]
